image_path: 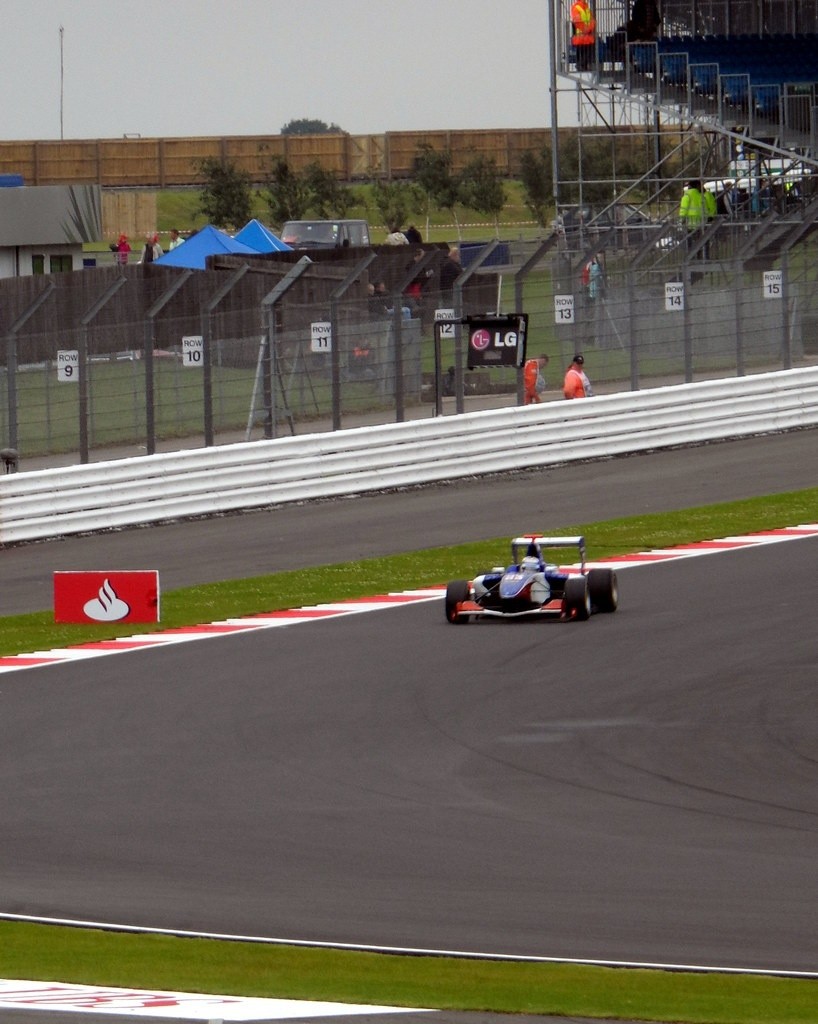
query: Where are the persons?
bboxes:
[169,229,185,254]
[367,282,389,321]
[683,175,808,244]
[582,253,606,306]
[373,281,394,310]
[678,180,709,276]
[402,248,435,335]
[383,227,409,245]
[518,544,546,571]
[141,232,165,264]
[116,234,131,266]
[440,247,468,309]
[403,222,422,244]
[564,355,593,399]
[522,355,548,405]
[349,334,379,377]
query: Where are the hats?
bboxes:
[573,356,584,364]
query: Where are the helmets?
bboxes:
[520,556,541,572]
